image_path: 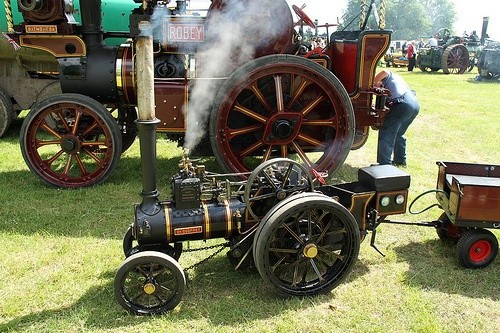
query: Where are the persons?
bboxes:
[407,40,417,71]
[303,36,327,50]
[429,30,479,50]
[402,38,430,60]
[372,70,420,167]
[390,42,395,53]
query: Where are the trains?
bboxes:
[112,33,500,316]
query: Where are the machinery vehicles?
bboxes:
[383,15,494,74]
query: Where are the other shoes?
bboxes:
[371,163,392,166]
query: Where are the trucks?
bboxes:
[0,0,141,140]
